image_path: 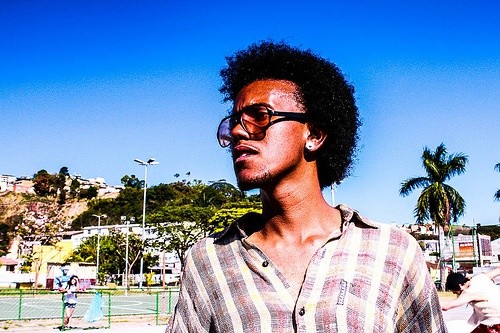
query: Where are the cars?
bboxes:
[105,267,180,287]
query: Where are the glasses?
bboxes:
[217,104,304,147]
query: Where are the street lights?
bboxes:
[92,214,107,284]
[134,157,155,288]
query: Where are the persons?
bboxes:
[165,41,448,333]
[62,275,79,329]
[441,268,500,333]
